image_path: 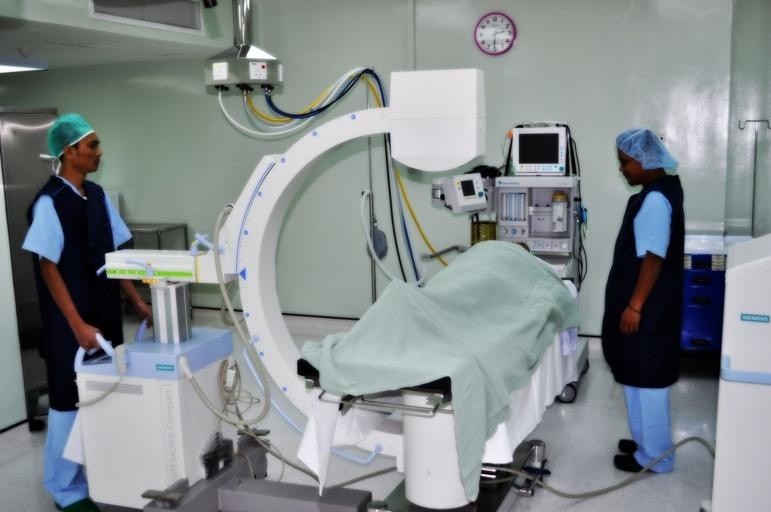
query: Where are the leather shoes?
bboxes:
[56,496,102,511]
[614,454,656,473]
[618,439,637,452]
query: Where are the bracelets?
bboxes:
[629,304,642,316]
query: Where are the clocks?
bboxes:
[474,10,519,56]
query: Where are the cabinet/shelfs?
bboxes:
[125,216,192,252]
[490,178,585,274]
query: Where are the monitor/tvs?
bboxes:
[511,127,567,176]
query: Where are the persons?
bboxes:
[600,130,684,473]
[22,114,153,510]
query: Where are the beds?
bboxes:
[315,253,589,497]
